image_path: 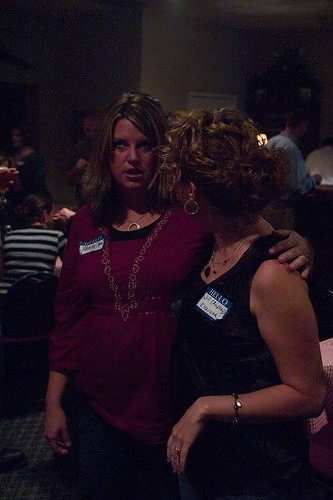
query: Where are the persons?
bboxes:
[0,193,69,295]
[304,134,333,186]
[0,116,93,228]
[44,90,320,500]
[264,105,324,232]
[153,103,326,500]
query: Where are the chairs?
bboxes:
[0,271,59,419]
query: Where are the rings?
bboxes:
[175,449,180,456]
[303,253,310,261]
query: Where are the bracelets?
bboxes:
[232,392,244,425]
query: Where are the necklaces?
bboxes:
[124,210,147,231]
[204,210,257,277]
